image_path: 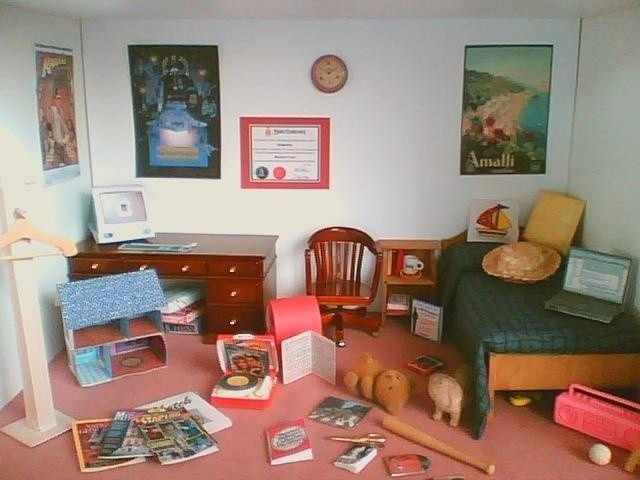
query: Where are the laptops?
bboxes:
[542,246,633,323]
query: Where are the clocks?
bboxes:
[311,55,348,93]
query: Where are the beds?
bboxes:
[441,225,640,425]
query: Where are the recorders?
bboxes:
[553,384,640,453]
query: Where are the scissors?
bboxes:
[331,433,387,447]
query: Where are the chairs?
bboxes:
[304,226,384,347]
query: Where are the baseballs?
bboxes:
[589,442,610,465]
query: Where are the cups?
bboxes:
[403,255,424,275]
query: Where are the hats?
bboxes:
[482,241,562,283]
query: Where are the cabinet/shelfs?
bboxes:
[67,232,278,345]
[377,238,442,327]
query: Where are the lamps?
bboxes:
[0,125,80,448]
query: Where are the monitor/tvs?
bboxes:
[86,183,155,245]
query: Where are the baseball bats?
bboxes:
[382,416,495,476]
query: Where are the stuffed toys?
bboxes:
[344,350,417,415]
[427,361,475,427]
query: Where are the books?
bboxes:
[332,444,378,474]
[387,309,410,316]
[386,291,411,310]
[381,451,427,478]
[306,395,373,431]
[265,416,316,467]
[279,328,337,386]
[71,389,231,474]
